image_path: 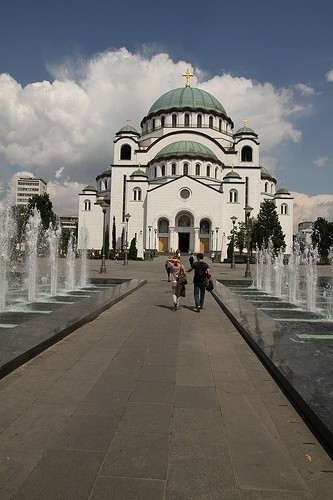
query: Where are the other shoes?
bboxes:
[200,305,202,309]
[174,303,177,311]
[177,305,181,309]
[197,306,201,311]
[168,280,170,282]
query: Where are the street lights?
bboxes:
[230,215,237,268]
[123,212,132,265]
[215,227,220,260]
[99,201,110,272]
[148,225,152,261]
[211,230,215,249]
[154,229,158,249]
[243,205,254,277]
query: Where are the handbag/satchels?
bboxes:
[206,279,214,292]
[179,267,187,285]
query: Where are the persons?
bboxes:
[174,248,215,266]
[185,253,211,311]
[167,256,188,311]
[165,256,173,282]
